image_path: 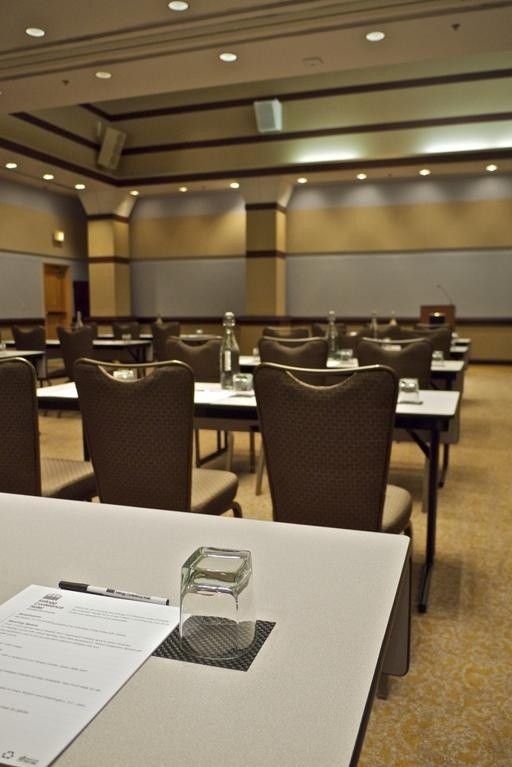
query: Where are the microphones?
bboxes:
[436,283,453,306]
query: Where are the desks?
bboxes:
[1,492,412,767]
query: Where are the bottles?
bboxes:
[219,310,241,389]
[75,311,83,329]
[389,309,396,327]
[155,312,163,324]
[324,310,339,362]
[369,310,378,329]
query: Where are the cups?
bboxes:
[430,350,443,365]
[113,368,139,381]
[337,347,353,364]
[231,372,254,392]
[397,377,419,403]
[177,546,258,662]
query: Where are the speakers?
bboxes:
[254,99,282,132]
[97,127,127,170]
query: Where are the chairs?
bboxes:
[0,303,474,615]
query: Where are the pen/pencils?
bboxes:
[58,581,168,606]
